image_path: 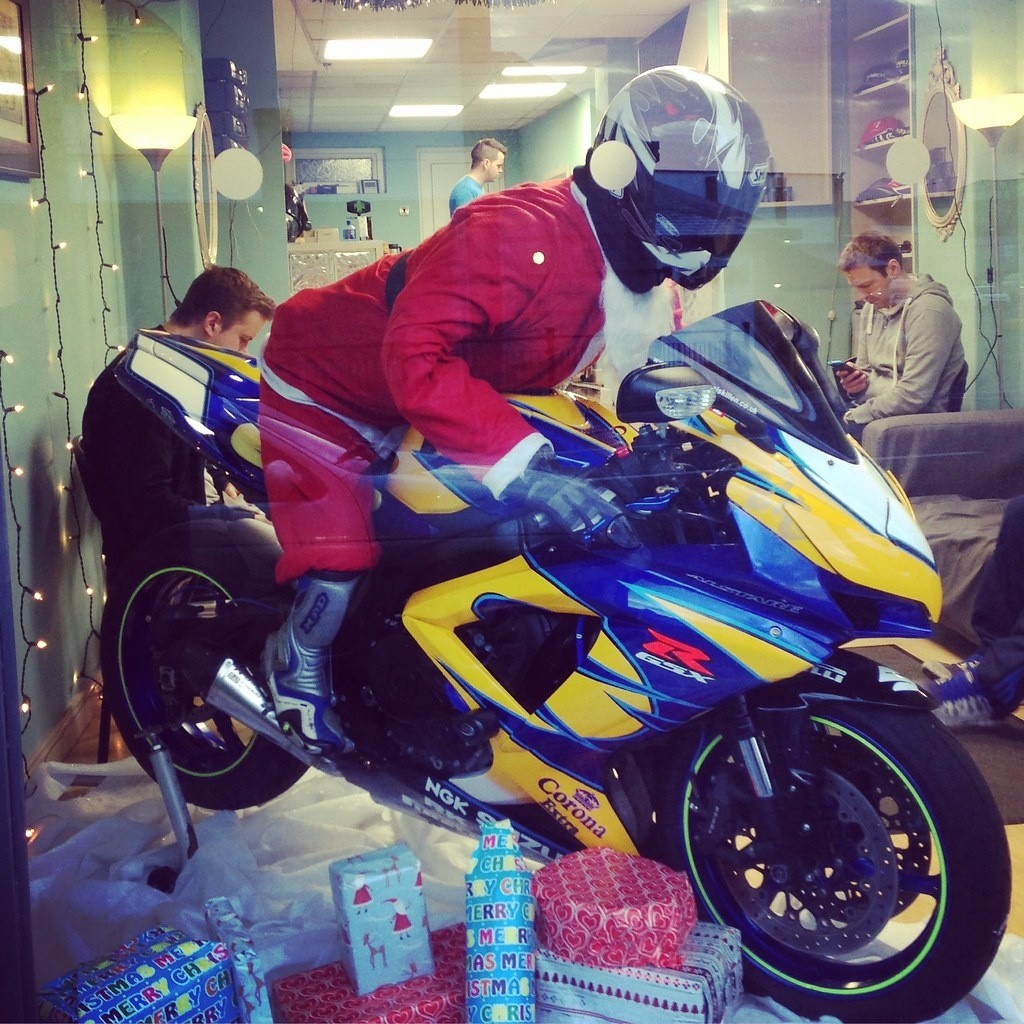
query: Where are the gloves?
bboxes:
[503,459,631,553]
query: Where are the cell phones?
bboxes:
[828,360,862,380]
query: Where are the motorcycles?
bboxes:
[94,295,1017,1024]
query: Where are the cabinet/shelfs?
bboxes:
[846,1,914,278]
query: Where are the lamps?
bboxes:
[951,93,1024,409]
[108,113,198,324]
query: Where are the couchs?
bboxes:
[863,409,1024,646]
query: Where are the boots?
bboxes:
[264,577,357,757]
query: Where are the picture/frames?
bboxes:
[0,0,40,178]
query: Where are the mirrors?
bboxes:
[924,92,956,218]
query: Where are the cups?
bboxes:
[754,158,792,202]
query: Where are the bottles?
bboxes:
[343,221,356,240]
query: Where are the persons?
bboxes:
[450,138,507,219]
[920,495,1024,722]
[262,68,773,746]
[828,232,970,428]
[82,263,277,591]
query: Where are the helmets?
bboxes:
[594,67,773,290]
